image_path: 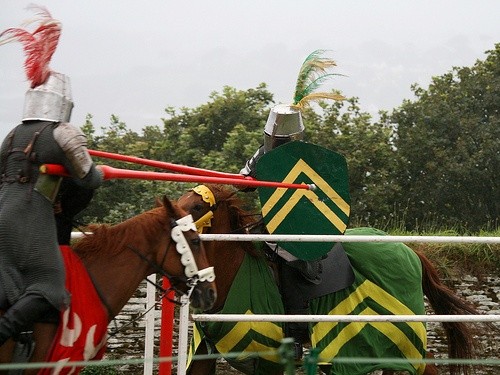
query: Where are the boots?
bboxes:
[286,301,308,358]
[0,293,49,349]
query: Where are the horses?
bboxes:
[0,194,217,375]
[177,183,500,375]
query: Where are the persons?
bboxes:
[0,5,103,345]
[233,50,348,359]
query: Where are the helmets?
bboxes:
[20,70,75,123]
[264,104,305,154]
